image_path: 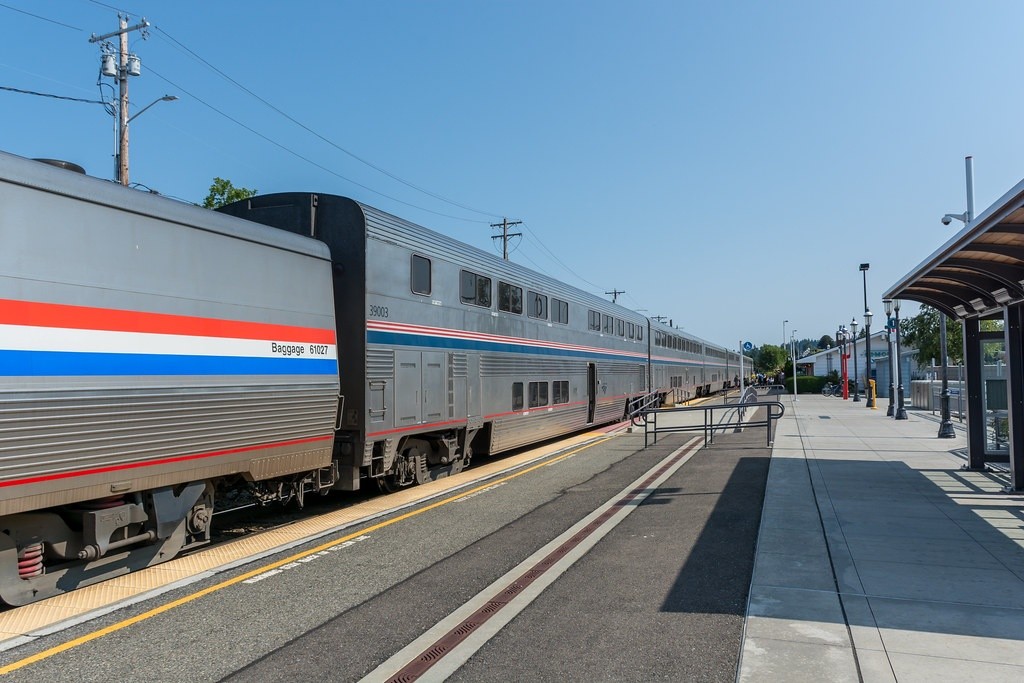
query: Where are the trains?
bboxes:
[0,150,754,607]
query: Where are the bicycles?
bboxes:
[822,380,843,397]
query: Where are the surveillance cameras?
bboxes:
[942,217,951,225]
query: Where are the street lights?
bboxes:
[120,95,179,186]
[783,319,797,359]
[850,317,861,401]
[836,324,848,377]
[882,299,908,419]
[859,263,873,407]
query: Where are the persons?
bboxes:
[734,374,738,387]
[773,370,783,389]
[744,372,768,390]
